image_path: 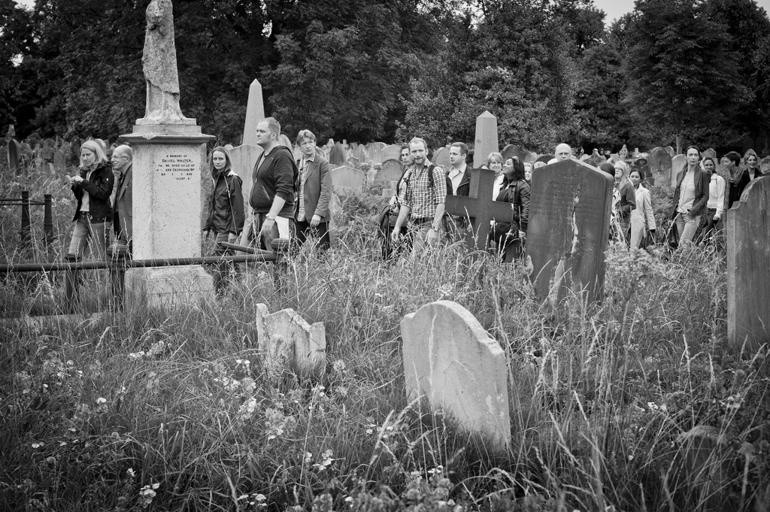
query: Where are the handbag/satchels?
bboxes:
[390,213,409,227]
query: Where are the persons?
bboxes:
[387,135,770,256]
[68,140,114,261]
[248,116,300,251]
[292,128,334,254]
[202,146,245,295]
[111,145,132,260]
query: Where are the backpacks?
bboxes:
[428,164,453,196]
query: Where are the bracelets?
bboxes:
[266,214,275,221]
